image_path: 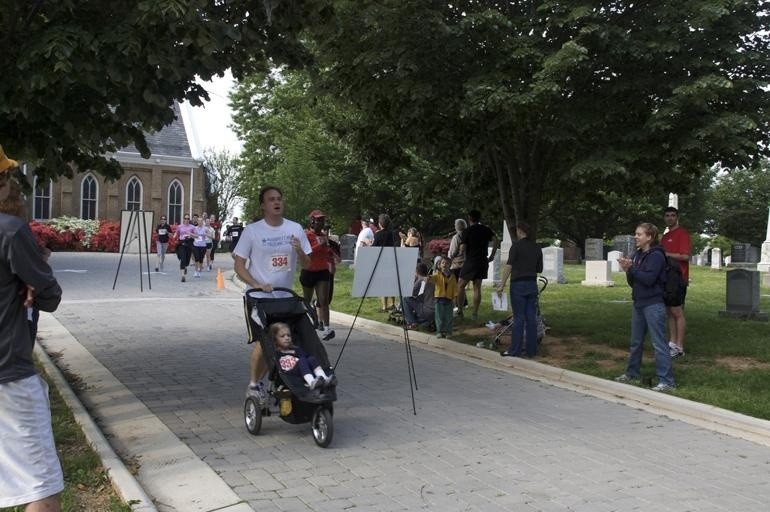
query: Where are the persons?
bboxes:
[0,145,65,512]
[223,225,232,237]
[226,217,244,253]
[368,220,376,230]
[240,223,243,229]
[402,263,436,332]
[367,214,401,313]
[485,315,513,331]
[615,223,675,391]
[232,186,312,411]
[202,211,208,258]
[496,220,543,357]
[399,229,410,246]
[447,219,469,312]
[355,218,374,268]
[454,210,500,321]
[210,214,222,264]
[313,223,341,331]
[153,215,173,272]
[425,257,461,338]
[205,218,215,271]
[269,322,338,389]
[418,232,425,260]
[189,213,199,266]
[405,228,419,246]
[661,207,692,358]
[349,217,362,236]
[173,214,199,282]
[193,216,211,278]
[299,210,335,341]
[433,255,443,273]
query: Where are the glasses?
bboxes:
[184,218,190,220]
[161,219,167,220]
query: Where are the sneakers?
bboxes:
[668,345,686,359]
[650,382,678,392]
[246,383,269,408]
[308,375,325,392]
[454,312,465,321]
[321,329,335,341]
[613,373,641,384]
[324,374,338,388]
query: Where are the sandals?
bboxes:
[403,324,418,330]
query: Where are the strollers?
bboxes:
[243,287,337,447]
[488,276,547,351]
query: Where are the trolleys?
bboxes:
[564,247,582,264]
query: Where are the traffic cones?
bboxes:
[217,270,226,290]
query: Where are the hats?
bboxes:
[360,215,370,222]
[310,209,326,219]
[0,142,19,173]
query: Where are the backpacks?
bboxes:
[642,248,684,306]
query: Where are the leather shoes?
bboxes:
[499,350,516,356]
